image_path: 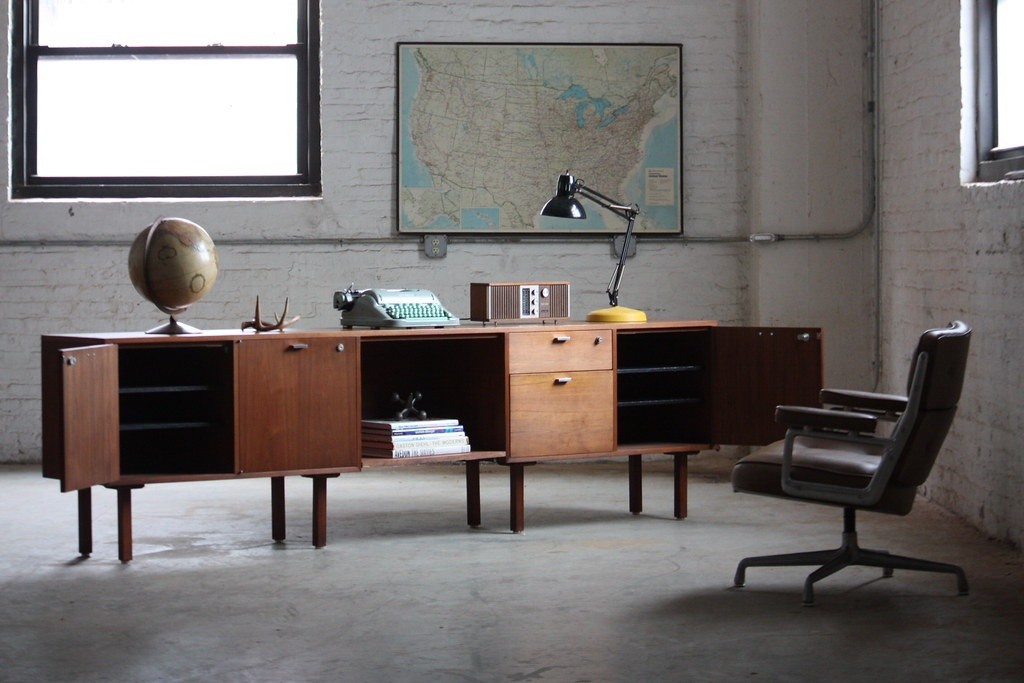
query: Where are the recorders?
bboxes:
[469,282,571,322]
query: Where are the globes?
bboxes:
[128,214,220,335]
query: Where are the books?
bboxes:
[360,417,471,459]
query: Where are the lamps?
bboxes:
[540,169,647,323]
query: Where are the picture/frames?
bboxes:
[395,41,684,234]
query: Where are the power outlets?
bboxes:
[423,234,446,258]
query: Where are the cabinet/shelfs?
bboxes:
[37,321,824,565]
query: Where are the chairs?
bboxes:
[730,321,973,606]
